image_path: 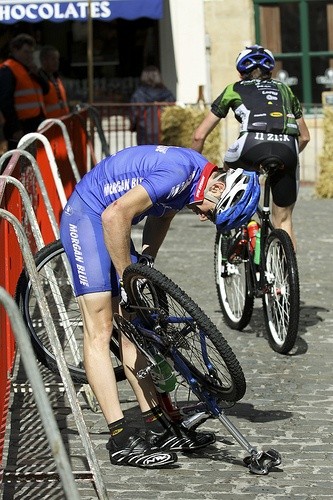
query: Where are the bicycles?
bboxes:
[13,237,286,477]
[212,157,301,354]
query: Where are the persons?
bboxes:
[0,34,50,173]
[194,44,311,303]
[128,66,176,146]
[36,45,70,118]
[60,145,262,470]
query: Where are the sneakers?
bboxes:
[143,420,216,450]
[227,226,250,264]
[107,433,178,468]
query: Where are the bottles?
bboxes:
[145,344,177,393]
[247,222,259,249]
[253,228,262,265]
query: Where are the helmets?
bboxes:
[213,168,261,230]
[235,43,277,73]
